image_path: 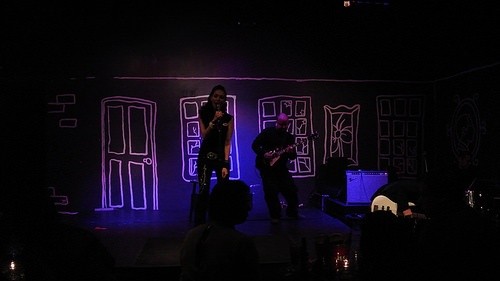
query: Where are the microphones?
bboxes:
[217,104,221,124]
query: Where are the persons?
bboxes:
[179,180,258,281]
[252,113,306,224]
[193,85,234,226]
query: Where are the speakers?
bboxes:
[336,169,393,206]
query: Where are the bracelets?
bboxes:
[209,120,216,129]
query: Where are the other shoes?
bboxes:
[287,215,304,220]
[272,218,279,223]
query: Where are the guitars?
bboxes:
[256,130,320,170]
